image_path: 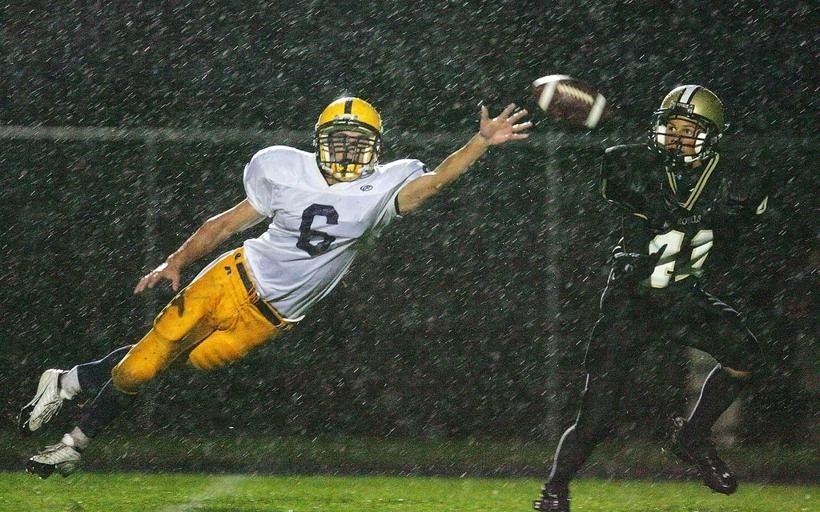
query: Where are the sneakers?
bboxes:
[17,370,75,436]
[25,441,86,479]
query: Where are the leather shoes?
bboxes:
[531,483,571,511]
[671,415,738,494]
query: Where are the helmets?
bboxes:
[650,85,729,171]
[313,96,387,183]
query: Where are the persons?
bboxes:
[530,83,772,512]
[17,95,535,482]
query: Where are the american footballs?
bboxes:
[522,74,617,133]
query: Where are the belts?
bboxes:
[235,252,304,333]
